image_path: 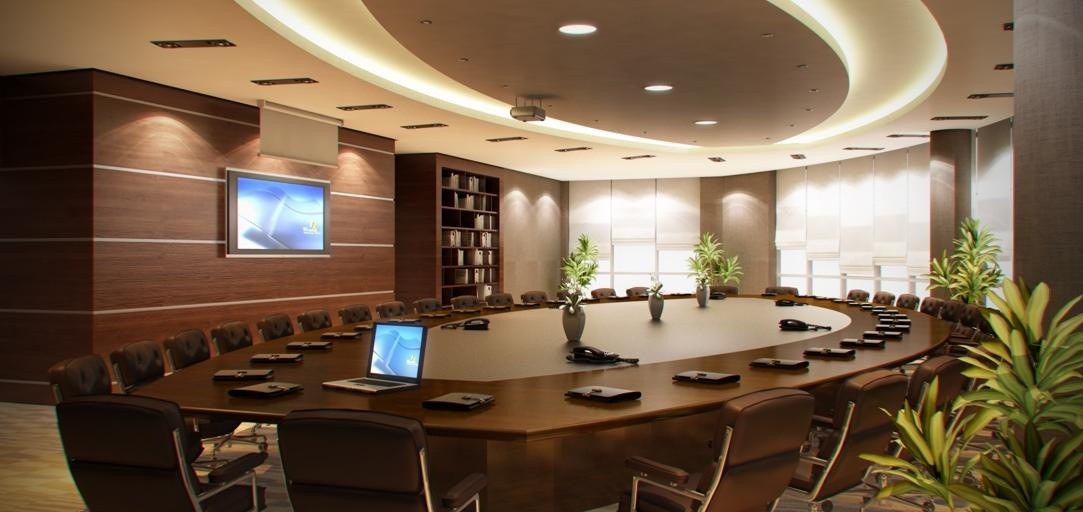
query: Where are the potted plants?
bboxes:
[687,233,742,307]
[558,235,598,340]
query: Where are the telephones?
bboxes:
[779,319,808,330]
[464,318,489,330]
[777,300,794,306]
[710,292,726,299]
[566,347,620,363]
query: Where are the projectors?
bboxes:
[510,106,545,122]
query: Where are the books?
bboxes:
[803,305,911,357]
[582,293,872,306]
[353,300,566,331]
[443,171,500,301]
[214,332,362,400]
[565,385,641,403]
[422,392,496,412]
[672,371,741,385]
[748,358,809,370]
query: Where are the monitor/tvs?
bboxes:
[223,167,330,258]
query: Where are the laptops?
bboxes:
[321,322,427,395]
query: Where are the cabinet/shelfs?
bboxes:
[396,153,505,313]
